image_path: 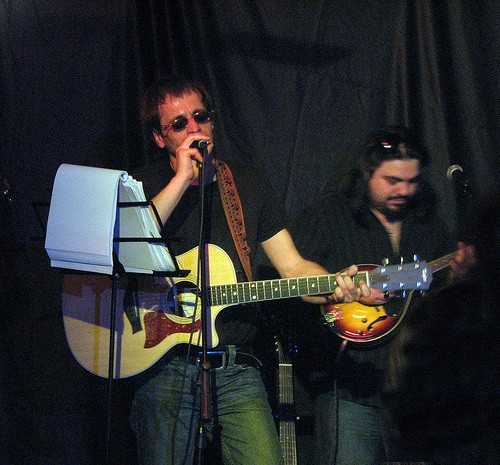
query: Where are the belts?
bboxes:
[183,344,263,373]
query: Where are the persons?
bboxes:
[126,73,371,465]
[287,126,481,465]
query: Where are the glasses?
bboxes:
[159,109,214,133]
[370,140,429,157]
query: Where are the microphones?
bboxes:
[191,141,207,149]
[447,165,475,194]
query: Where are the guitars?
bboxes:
[322,246,475,350]
[60,243,432,379]
